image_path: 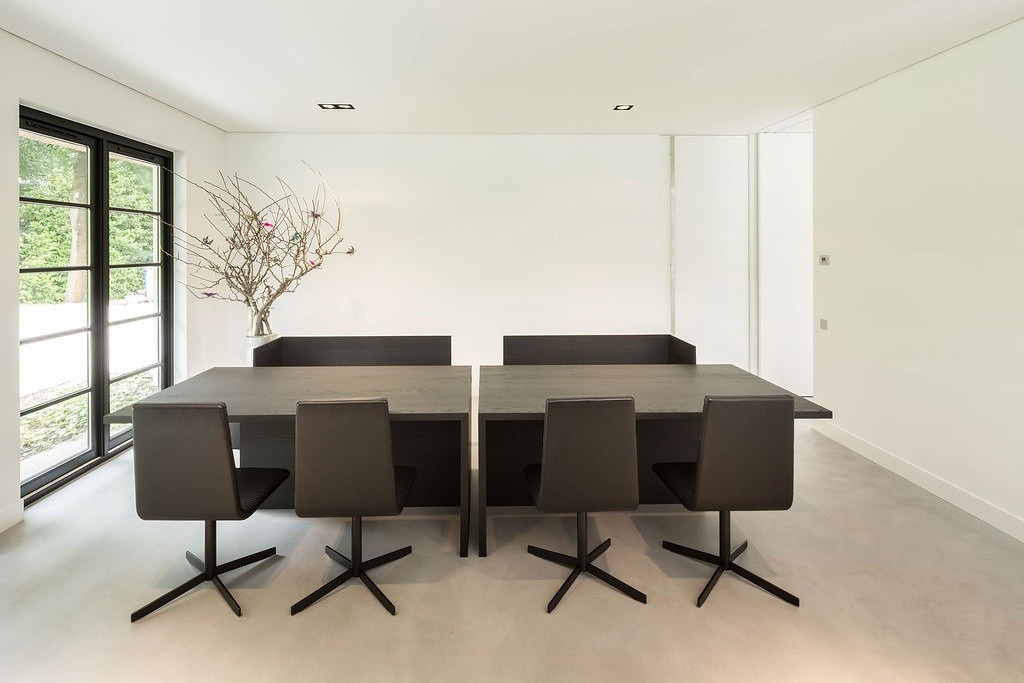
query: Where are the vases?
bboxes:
[245,334,270,367]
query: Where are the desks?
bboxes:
[103,366,471,558]
[478,364,833,550]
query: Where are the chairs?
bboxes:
[130,401,276,622]
[661,395,800,608]
[527,396,647,616]
[282,398,413,616]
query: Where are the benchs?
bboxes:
[502,335,697,366]
[253,333,452,366]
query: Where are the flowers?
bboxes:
[148,163,357,334]
